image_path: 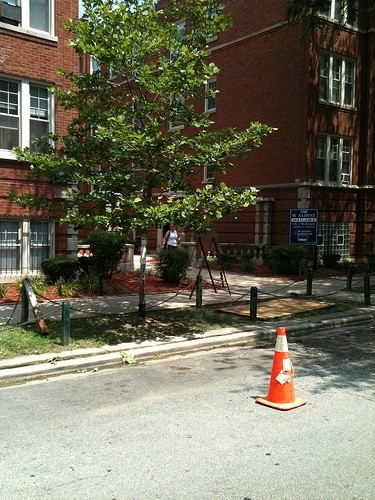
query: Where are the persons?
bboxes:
[162,223,183,249]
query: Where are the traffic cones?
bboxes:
[256,327,307,411]
